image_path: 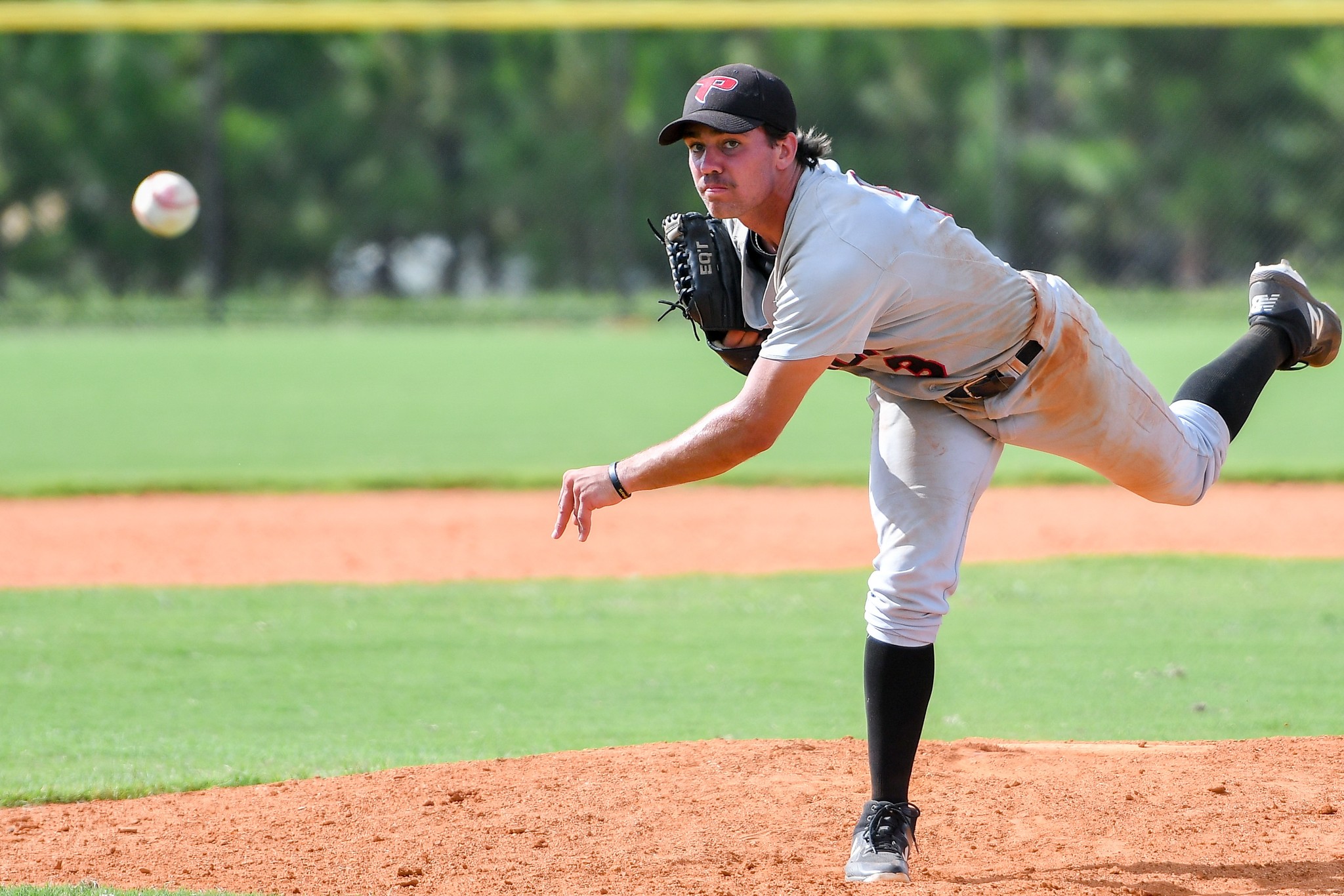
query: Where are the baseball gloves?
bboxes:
[662,212,772,376]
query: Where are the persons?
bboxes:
[551,66,1342,886]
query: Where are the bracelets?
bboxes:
[607,460,633,501]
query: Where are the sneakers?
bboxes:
[844,800,921,882]
[1246,258,1343,371]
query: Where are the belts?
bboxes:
[941,342,1040,401]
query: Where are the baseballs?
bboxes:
[131,170,198,238]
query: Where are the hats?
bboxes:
[659,61,797,147]
